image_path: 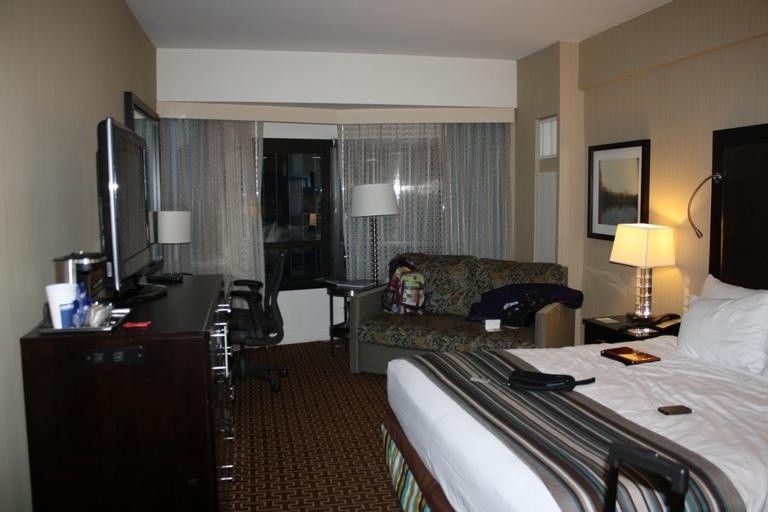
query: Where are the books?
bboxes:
[334,280,377,288]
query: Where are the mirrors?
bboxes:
[124,91,162,246]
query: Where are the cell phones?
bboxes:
[658,406,691,414]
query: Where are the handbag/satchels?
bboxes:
[506,370,596,392]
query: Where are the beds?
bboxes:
[380,336,767,512]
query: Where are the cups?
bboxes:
[59,304,75,328]
[46,283,78,329]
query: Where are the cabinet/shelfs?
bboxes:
[20,273,235,512]
[327,279,390,357]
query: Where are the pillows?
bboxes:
[698,275,767,298]
[676,294,767,373]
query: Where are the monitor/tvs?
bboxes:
[95,116,168,307]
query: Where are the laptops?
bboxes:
[602,347,660,366]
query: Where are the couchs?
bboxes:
[349,253,575,374]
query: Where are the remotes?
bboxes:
[148,277,182,281]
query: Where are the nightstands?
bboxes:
[582,313,681,345]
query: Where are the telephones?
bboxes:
[650,313,682,331]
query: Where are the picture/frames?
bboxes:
[587,139,650,241]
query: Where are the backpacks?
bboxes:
[380,266,427,317]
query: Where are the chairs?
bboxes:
[226,248,287,393]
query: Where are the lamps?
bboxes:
[155,213,192,275]
[349,183,400,284]
[687,172,722,240]
[609,221,676,319]
